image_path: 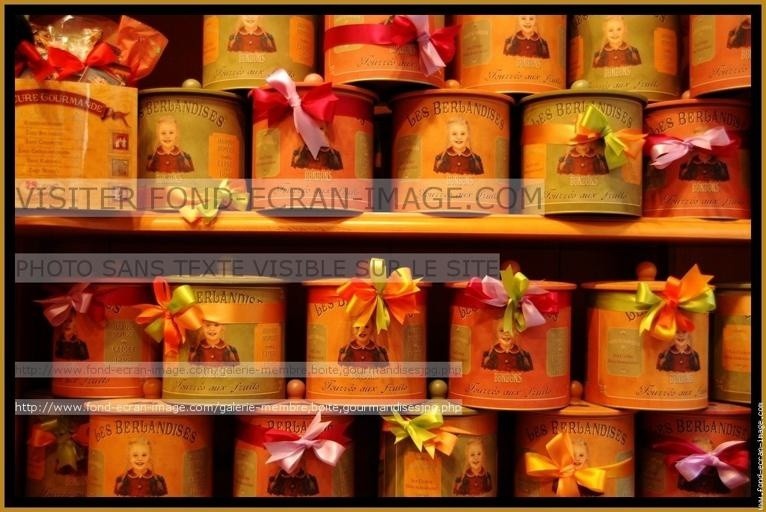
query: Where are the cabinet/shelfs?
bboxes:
[0,0,761,506]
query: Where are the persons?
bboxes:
[503,14,549,60]
[677,438,731,492]
[727,16,752,48]
[113,438,167,495]
[267,456,319,496]
[227,15,276,53]
[592,16,641,68]
[188,320,239,367]
[678,150,729,182]
[556,144,611,176]
[479,317,533,374]
[434,117,484,174]
[54,317,89,362]
[451,437,493,495]
[145,115,193,172]
[338,318,390,368]
[657,328,702,373]
[290,121,344,170]
[553,439,604,498]
